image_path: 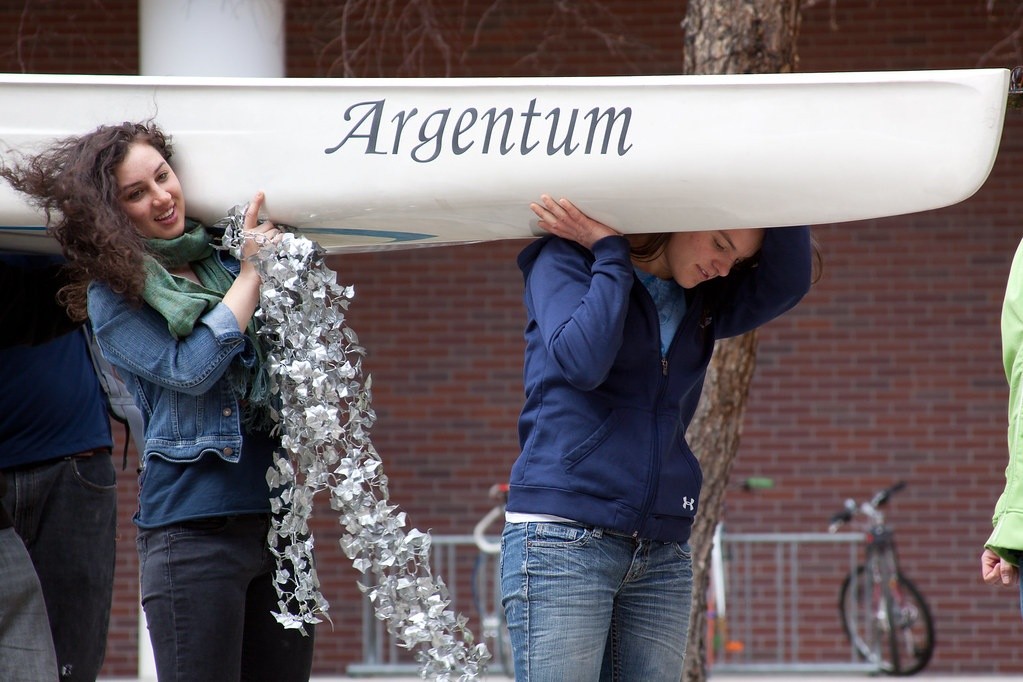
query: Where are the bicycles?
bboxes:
[826,478,940,678]
[470,472,510,682]
[704,474,773,673]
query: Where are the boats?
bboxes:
[0,62,1017,266]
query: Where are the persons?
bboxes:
[0,245,119,682]
[500,194,812,682]
[0,121,313,682]
[981,238,1023,620]
